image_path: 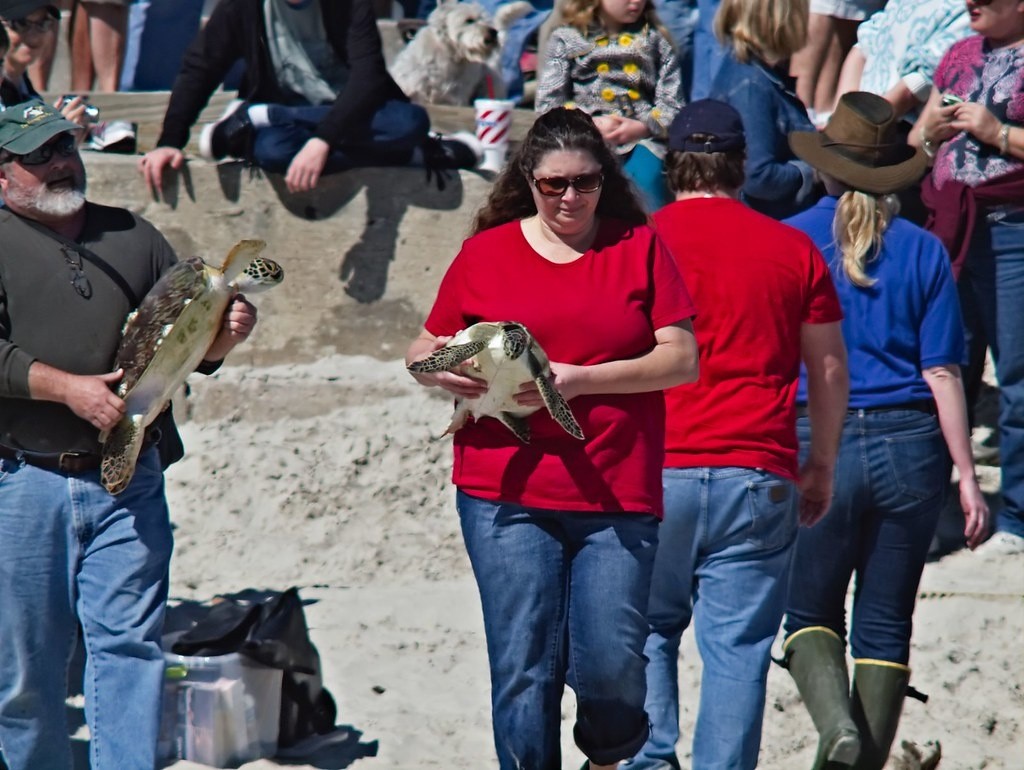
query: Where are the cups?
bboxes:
[473,98,514,170]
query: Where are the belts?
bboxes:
[795,403,812,418]
[0,433,155,474]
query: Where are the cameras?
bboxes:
[942,94,965,107]
[62,97,99,123]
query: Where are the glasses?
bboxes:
[0,16,55,33]
[528,170,605,196]
[61,244,93,299]
[0,133,75,166]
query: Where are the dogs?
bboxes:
[387,0,504,105]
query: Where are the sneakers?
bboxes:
[200,99,256,161]
[418,131,484,169]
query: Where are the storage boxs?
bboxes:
[158,598,284,769]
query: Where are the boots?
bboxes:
[782,625,860,770]
[851,656,929,770]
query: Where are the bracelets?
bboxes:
[921,128,934,156]
[1000,124,1010,153]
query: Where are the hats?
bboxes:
[0,99,82,155]
[669,99,744,153]
[788,92,929,195]
[0,0,61,20]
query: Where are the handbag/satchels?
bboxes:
[173,586,338,748]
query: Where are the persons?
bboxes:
[0,99,256,770]
[0,0,980,193]
[406,106,701,769]
[905,0,1024,558]
[617,98,851,769]
[780,90,990,769]
